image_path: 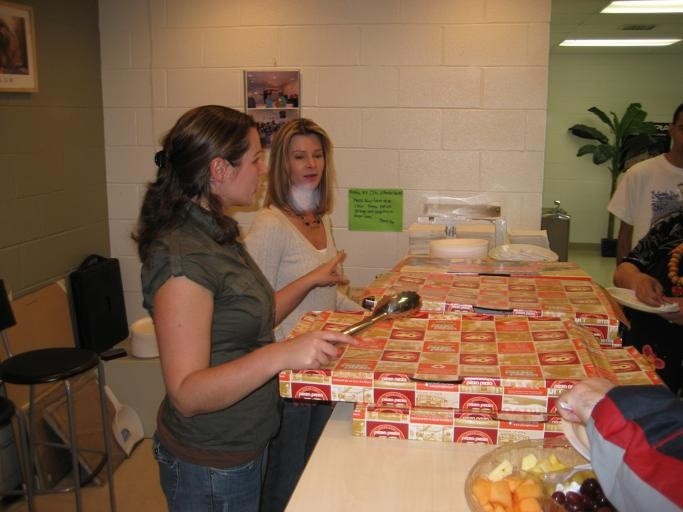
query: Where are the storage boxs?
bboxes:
[100,337,160,440]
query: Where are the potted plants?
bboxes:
[569,101,660,256]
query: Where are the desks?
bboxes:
[283,401,494,512]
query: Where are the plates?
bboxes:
[608,285,679,315]
[488,244,559,263]
[431,238,488,259]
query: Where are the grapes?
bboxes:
[552,478,615,512]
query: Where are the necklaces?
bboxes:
[297,211,322,229]
[667,242,683,297]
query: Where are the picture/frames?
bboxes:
[242,68,302,150]
[0,3,39,93]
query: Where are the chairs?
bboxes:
[0,398,34,512]
[0,278,116,512]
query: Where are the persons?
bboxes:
[132,105,359,512]
[556,376,683,512]
[242,119,366,512]
[278,93,285,108]
[266,93,271,108]
[613,209,683,395]
[256,121,282,145]
[606,103,683,267]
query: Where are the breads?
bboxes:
[471,455,565,512]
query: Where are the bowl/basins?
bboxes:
[467,441,617,511]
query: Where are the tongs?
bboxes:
[327,290,421,345]
